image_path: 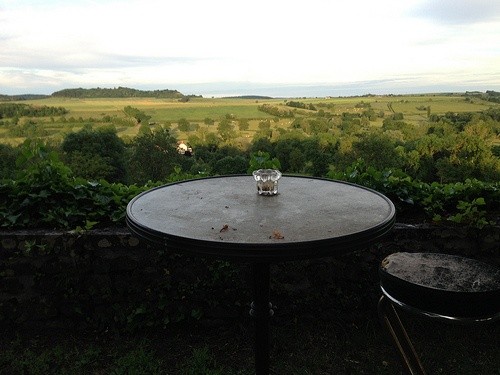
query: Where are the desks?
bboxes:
[126,175,397,375]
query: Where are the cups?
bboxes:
[252,168,281,195]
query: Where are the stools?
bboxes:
[377,253,500,375]
